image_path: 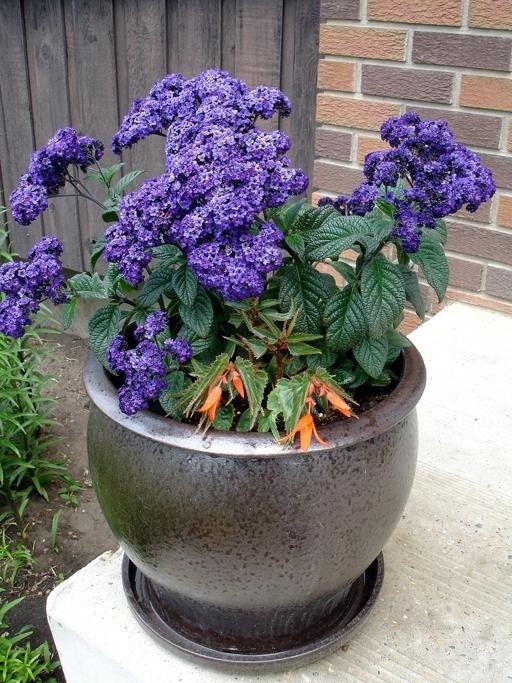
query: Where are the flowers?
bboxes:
[0,69,498,453]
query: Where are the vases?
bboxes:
[81,328,427,676]
